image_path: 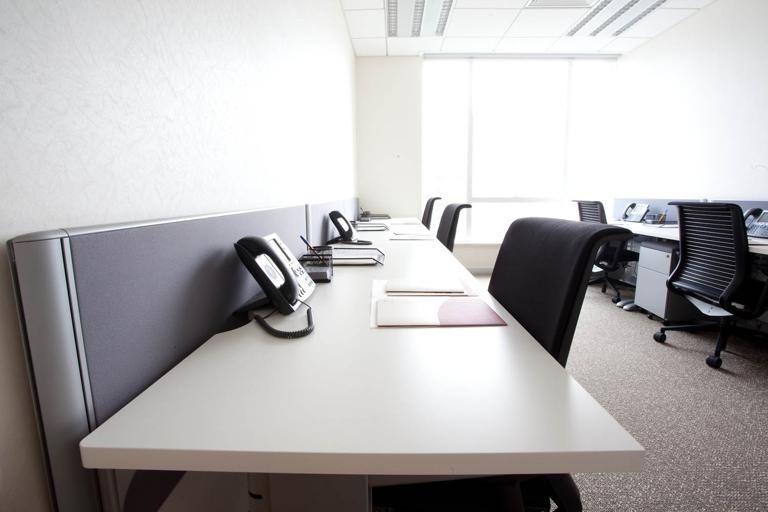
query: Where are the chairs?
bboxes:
[434,204,474,249]
[422,196,441,231]
[571,200,639,304]
[371,217,634,512]
[652,202,766,369]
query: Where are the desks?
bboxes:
[79,217,647,512]
[607,219,768,322]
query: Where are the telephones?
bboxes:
[233,232,317,315]
[743,207,768,238]
[328,210,358,241]
[621,201,650,222]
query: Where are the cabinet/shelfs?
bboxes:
[633,241,700,325]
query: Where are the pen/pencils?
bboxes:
[360,207,366,216]
[300,235,328,265]
[658,208,668,223]
